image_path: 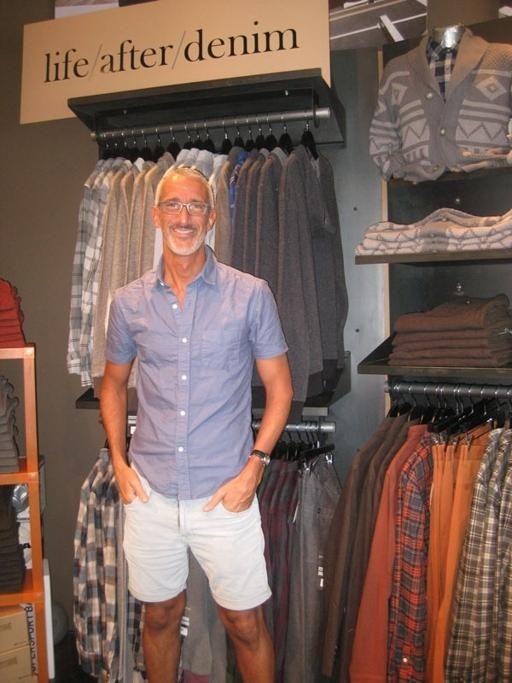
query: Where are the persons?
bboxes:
[101,163,296,682]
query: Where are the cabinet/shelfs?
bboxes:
[355,245,510,379]
[0,345,48,682]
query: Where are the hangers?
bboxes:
[383,377,511,440]
[277,421,317,465]
[94,104,324,160]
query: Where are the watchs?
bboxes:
[250,449,271,466]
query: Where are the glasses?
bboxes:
[162,200,209,215]
